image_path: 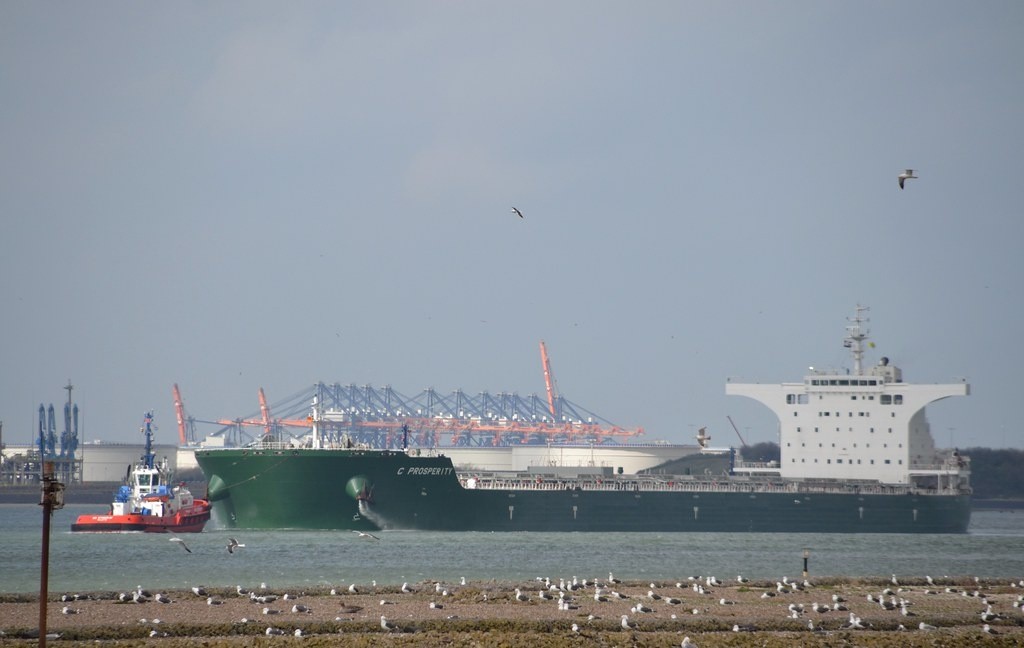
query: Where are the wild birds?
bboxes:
[511,207,524,219]
[695,427,710,446]
[356,481,375,504]
[512,572,737,648]
[59,529,311,639]
[733,575,938,633]
[897,169,918,189]
[330,572,467,632]
[946,576,1024,636]
[352,530,380,541]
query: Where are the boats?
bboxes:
[69,412,213,533]
[193,298,976,535]
